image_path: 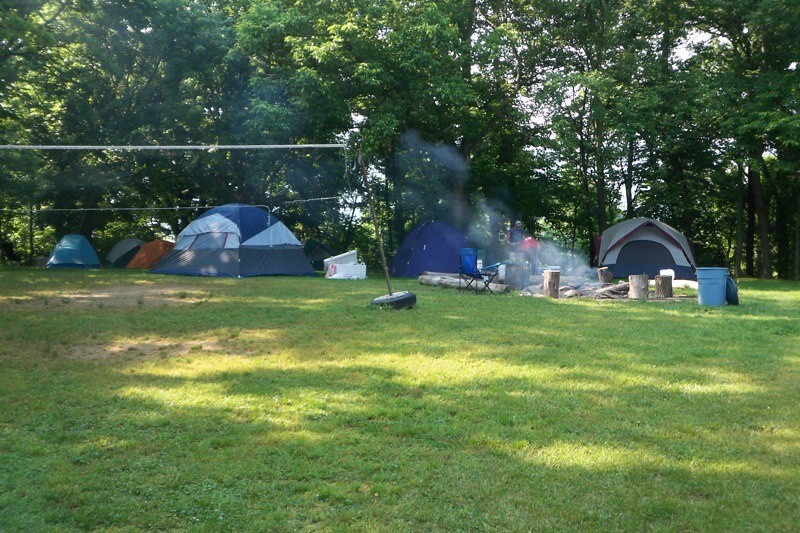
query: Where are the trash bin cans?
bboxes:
[695,267,729,306]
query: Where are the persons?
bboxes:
[504,220,527,265]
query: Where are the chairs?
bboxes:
[457,248,502,295]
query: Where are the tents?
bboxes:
[595,216,699,281]
[389,219,473,282]
[45,234,100,268]
[125,240,177,268]
[105,239,146,267]
[148,202,320,278]
